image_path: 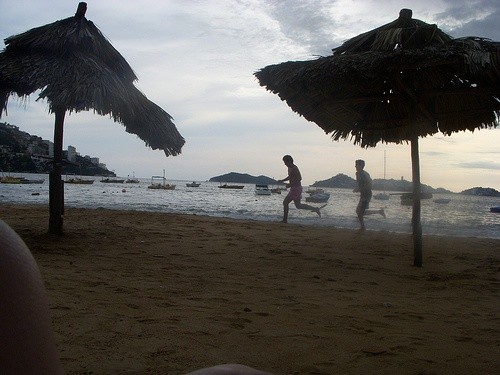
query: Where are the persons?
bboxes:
[352,159,386,232]
[276,154,321,224]
[0,217,273,375]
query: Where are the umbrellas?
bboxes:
[0,1,186,238]
[255,8,500,267]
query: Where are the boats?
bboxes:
[305,188,324,193]
[432,198,450,204]
[270,188,282,194]
[0,169,46,184]
[372,191,388,200]
[304,189,331,203]
[186,181,201,187]
[125,179,140,183]
[64,174,94,184]
[218,183,244,189]
[148,183,176,190]
[255,183,272,195]
[100,176,124,184]
[276,187,288,191]
